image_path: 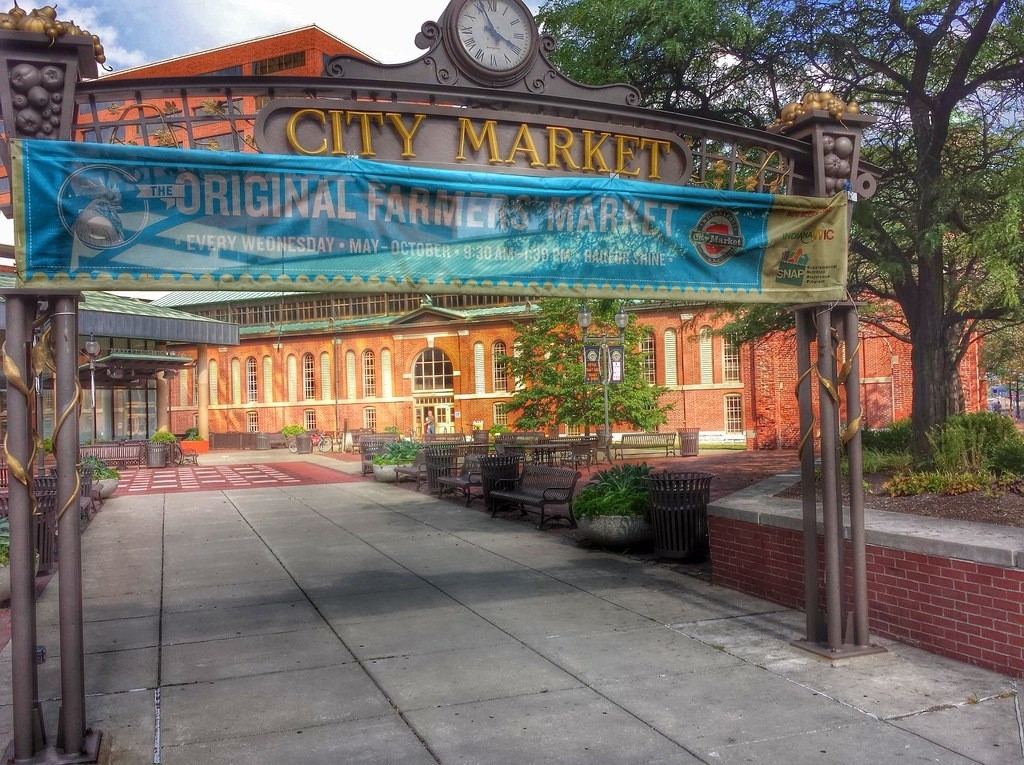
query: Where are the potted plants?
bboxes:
[152,430,176,465]
[567,462,655,552]
[0,517,39,602]
[372,441,424,483]
[79,454,121,498]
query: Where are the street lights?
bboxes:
[576,303,629,460]
[84,330,100,444]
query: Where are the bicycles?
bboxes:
[288,429,333,454]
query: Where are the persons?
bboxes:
[424,410,435,434]
[993,400,1003,415]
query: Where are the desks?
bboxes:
[522,444,570,468]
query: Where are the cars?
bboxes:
[986,383,1024,413]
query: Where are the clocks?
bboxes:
[442,0,541,89]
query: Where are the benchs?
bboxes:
[0,460,103,527]
[78,444,141,471]
[394,449,426,491]
[436,453,487,507]
[359,436,400,478]
[614,431,678,460]
[541,436,612,466]
[489,465,582,531]
[175,444,198,466]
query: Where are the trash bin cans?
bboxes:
[640,470,717,561]
[472,429,490,454]
[421,447,460,494]
[595,425,613,445]
[144,441,166,468]
[296,434,311,454]
[476,454,522,512]
[675,427,701,456]
[29,477,58,573]
[79,466,94,518]
